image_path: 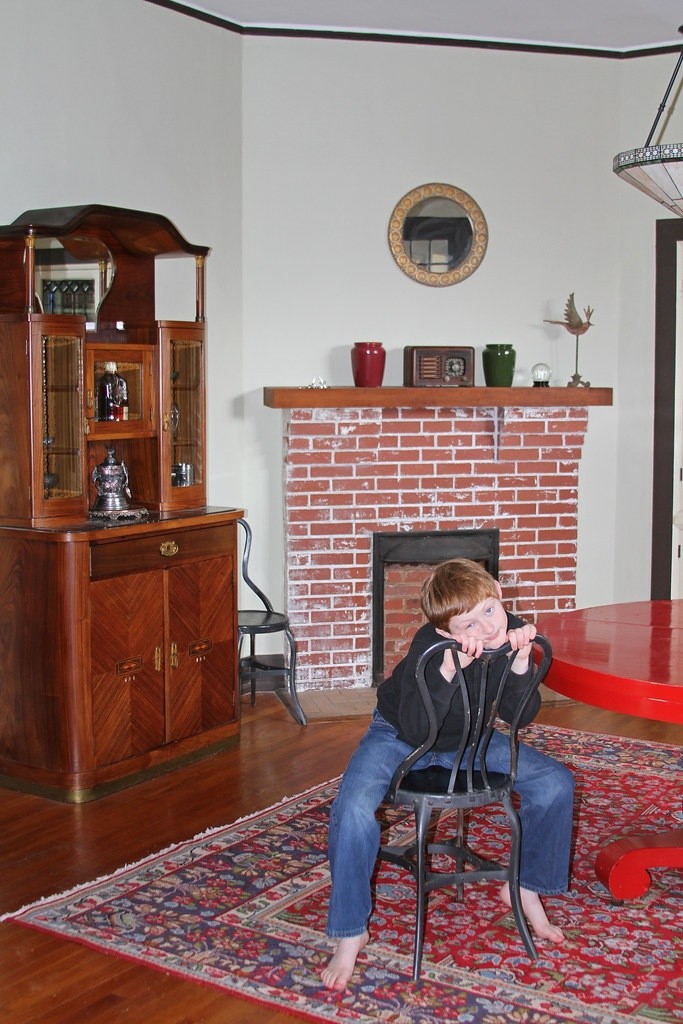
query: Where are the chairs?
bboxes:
[377,633,553,980]
[237,518,308,727]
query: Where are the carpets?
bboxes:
[0,717,683,1019]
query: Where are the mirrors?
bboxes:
[387,183,489,288]
[24,229,115,329]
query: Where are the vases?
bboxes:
[351,342,386,388]
[481,343,516,388]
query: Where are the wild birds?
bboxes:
[543,293,596,336]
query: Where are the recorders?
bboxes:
[404,346,473,388]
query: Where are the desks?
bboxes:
[528,599,683,899]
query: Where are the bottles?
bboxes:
[96,361,128,421]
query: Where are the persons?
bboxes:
[320,558,574,990]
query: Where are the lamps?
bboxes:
[613,26,683,219]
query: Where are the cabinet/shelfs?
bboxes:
[0,313,88,528]
[0,505,245,803]
[97,320,207,512]
[84,343,155,442]
